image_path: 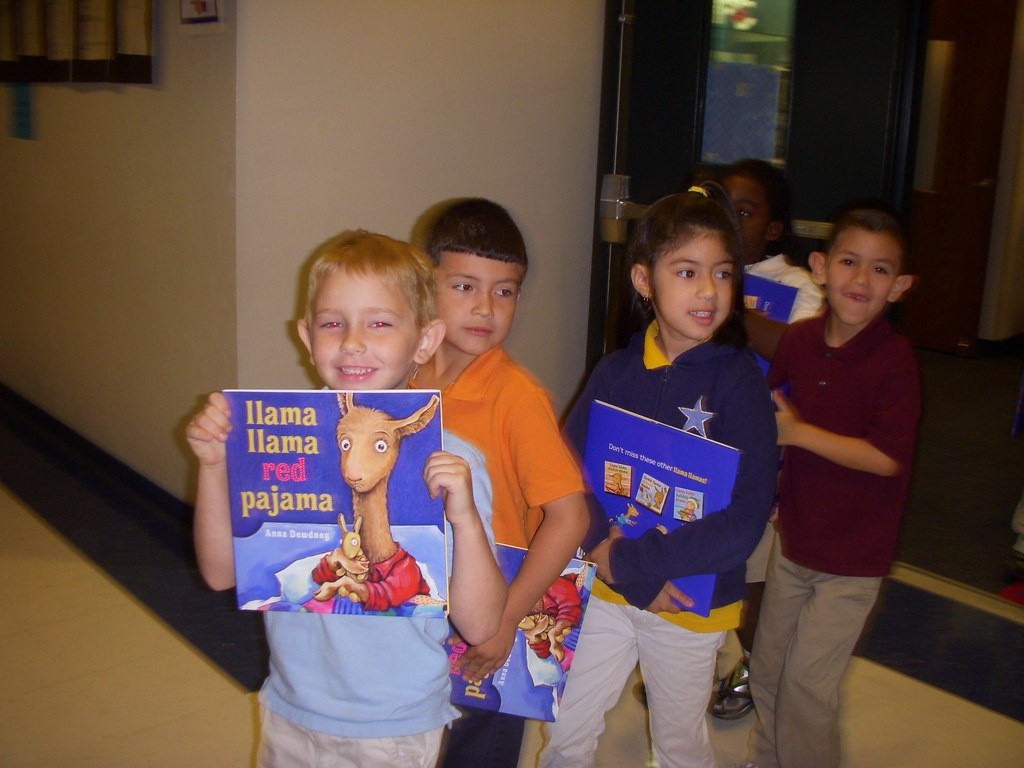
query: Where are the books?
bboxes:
[741,271,797,378]
[222,389,449,620]
[584,400,742,618]
[444,542,597,722]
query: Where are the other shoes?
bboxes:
[713,648,755,720]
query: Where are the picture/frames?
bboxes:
[178,0,219,25]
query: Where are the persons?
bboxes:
[711,158,827,720]
[187,229,510,768]
[742,209,920,768]
[413,197,591,768]
[535,178,775,767]
[997,496,1024,607]
